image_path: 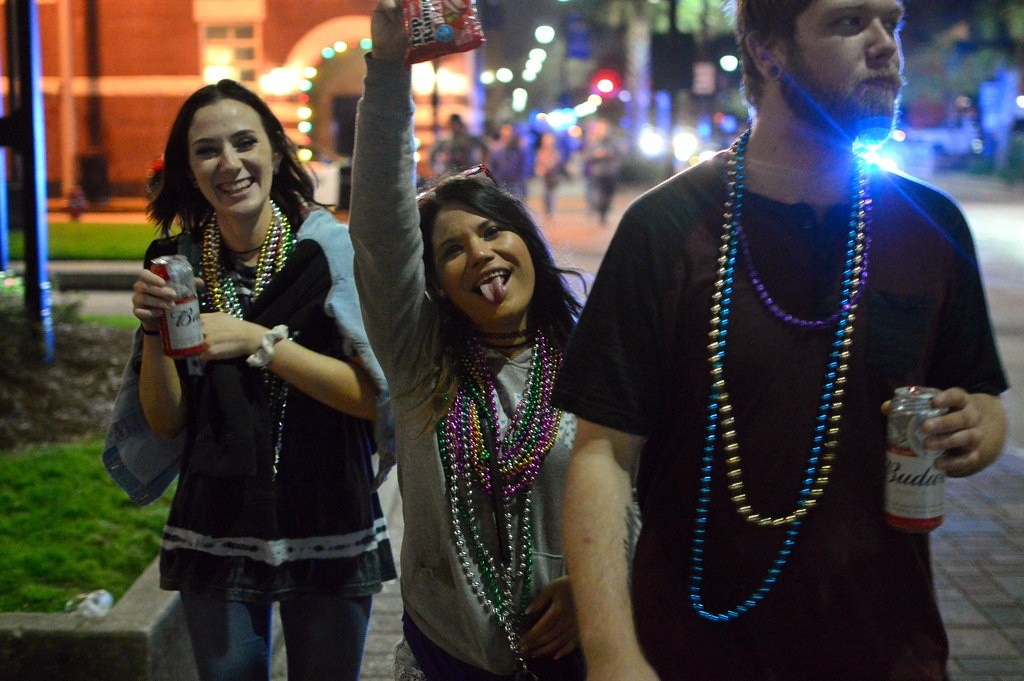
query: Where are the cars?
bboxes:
[892,110,980,157]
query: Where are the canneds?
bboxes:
[882,385,950,533]
[150,254,205,359]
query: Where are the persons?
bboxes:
[349,0,646,681]
[103,77,397,681]
[546,0,1009,681]
[429,113,624,223]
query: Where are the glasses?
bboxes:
[414,163,499,208]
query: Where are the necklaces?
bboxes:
[685,124,869,621]
[435,322,562,681]
[200,201,298,478]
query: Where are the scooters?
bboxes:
[587,174,616,223]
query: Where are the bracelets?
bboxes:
[249,325,291,367]
[141,324,160,337]
